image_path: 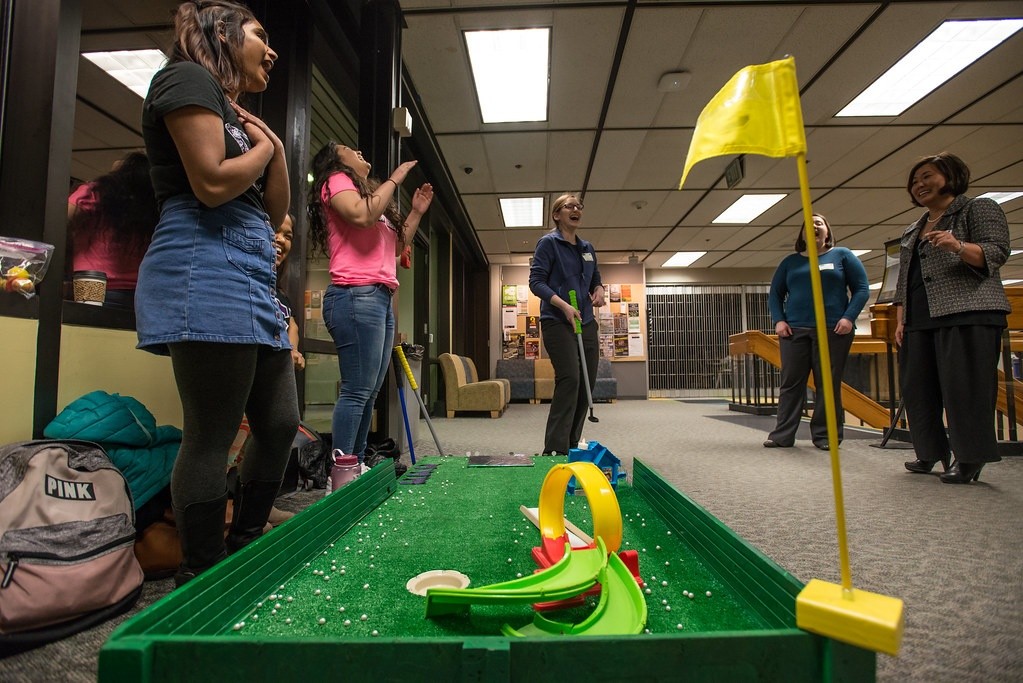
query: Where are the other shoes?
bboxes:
[263,507,294,534]
[763,439,780,448]
[819,443,829,449]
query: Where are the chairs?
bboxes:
[439,353,617,420]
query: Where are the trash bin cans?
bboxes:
[372,342,426,454]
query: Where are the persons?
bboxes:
[530,194,605,456]
[68,150,156,290]
[224,214,298,535]
[135,0,299,589]
[892,151,1011,483]
[763,213,871,451]
[305,138,435,496]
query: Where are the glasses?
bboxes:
[558,203,585,209]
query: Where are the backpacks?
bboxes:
[268,419,325,524]
[0,437,146,644]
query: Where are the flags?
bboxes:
[679,59,806,192]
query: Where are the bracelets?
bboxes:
[385,179,397,188]
[955,240,965,255]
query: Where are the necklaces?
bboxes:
[927,212,944,223]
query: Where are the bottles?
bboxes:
[332,448,361,491]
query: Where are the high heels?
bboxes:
[939,457,986,484]
[905,451,952,473]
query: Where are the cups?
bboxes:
[73,271,107,306]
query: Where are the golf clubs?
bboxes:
[391,348,416,464]
[395,345,445,456]
[568,288,599,423]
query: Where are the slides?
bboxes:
[423,535,608,619]
[497,550,648,638]
